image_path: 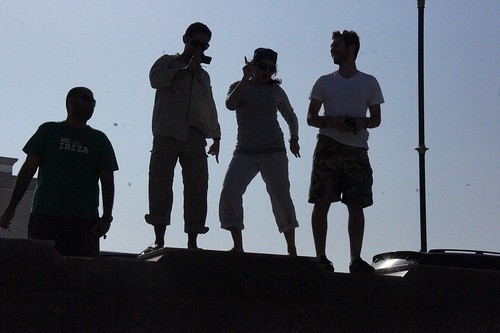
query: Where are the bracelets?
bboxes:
[185,63,191,71]
[99,214,113,222]
[212,136,221,140]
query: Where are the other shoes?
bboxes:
[350,257,374,273]
[318,255,334,272]
[138,246,155,259]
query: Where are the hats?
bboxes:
[253,48,277,65]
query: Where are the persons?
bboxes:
[137,22,221,255]
[305,29,384,273]
[0,84,118,255]
[219,46,301,256]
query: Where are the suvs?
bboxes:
[372,247,500,270]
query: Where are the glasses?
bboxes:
[341,30,356,44]
[72,94,96,107]
[253,63,276,74]
[186,34,210,50]
